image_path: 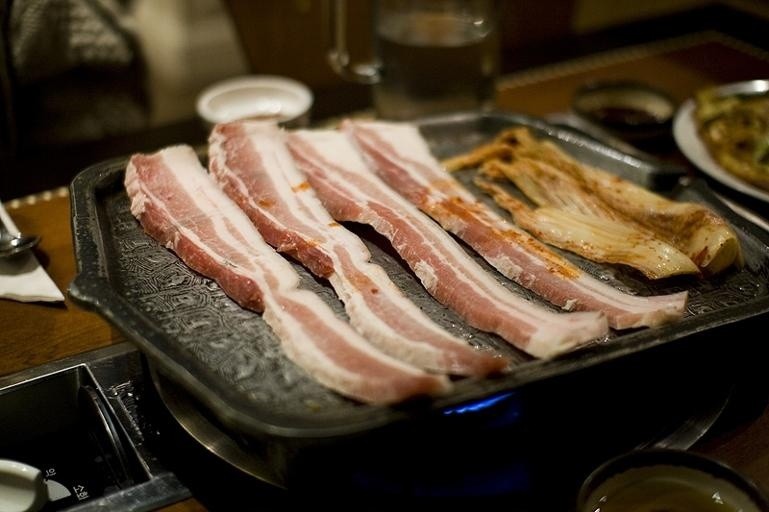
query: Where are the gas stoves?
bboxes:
[145,314,737,493]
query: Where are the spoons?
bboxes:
[325,0,386,88]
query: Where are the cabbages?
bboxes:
[441,126,745,280]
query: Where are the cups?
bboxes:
[325,11,500,122]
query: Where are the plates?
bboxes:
[669,69,769,213]
[66,109,769,438]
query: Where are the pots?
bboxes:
[576,446,769,512]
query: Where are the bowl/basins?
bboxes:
[191,74,317,138]
[568,77,678,142]
